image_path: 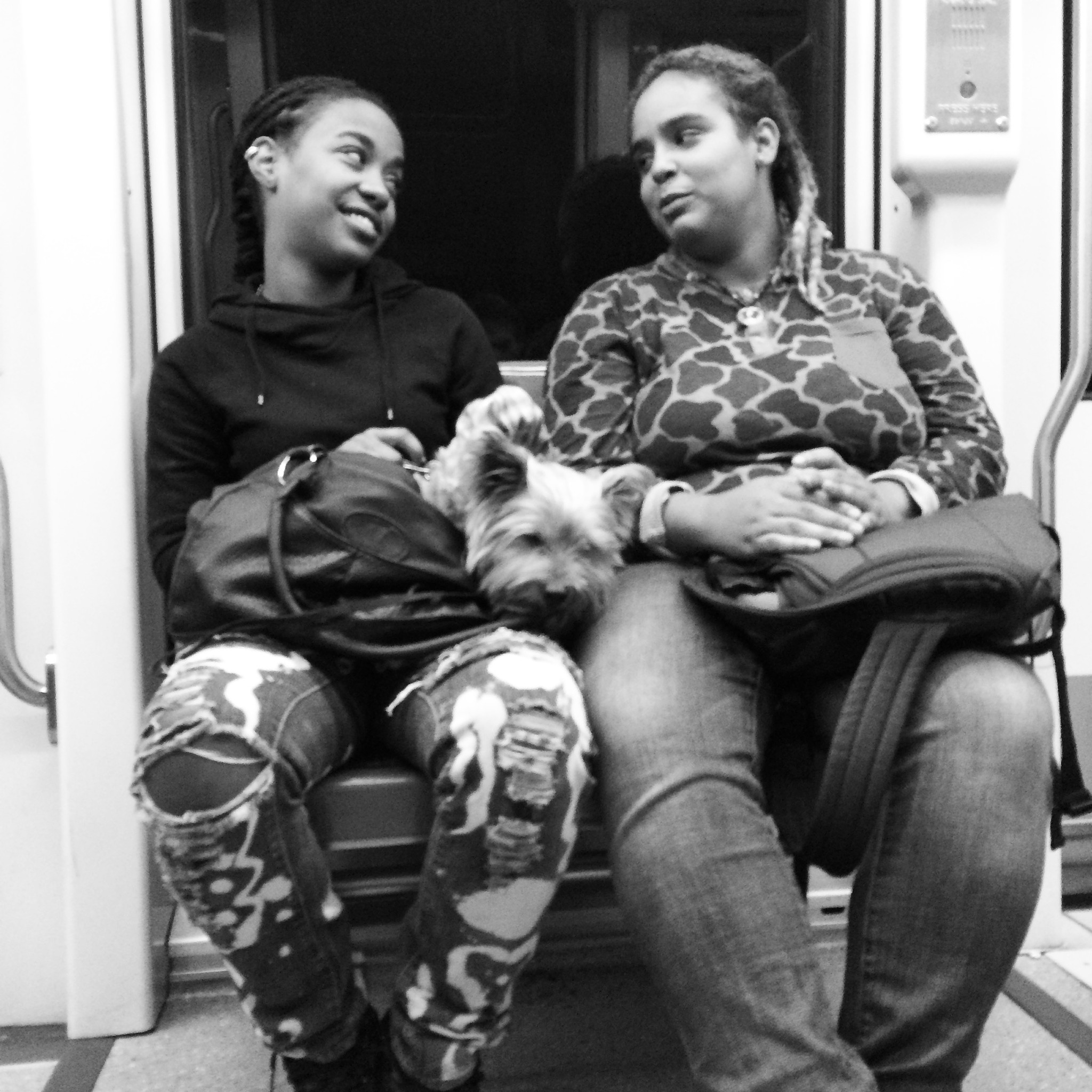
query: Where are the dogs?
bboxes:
[430,423,644,636]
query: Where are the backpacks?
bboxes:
[683,493,1063,880]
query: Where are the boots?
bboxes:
[281,1003,488,1091]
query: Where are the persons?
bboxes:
[538,41,1057,1092]
[127,72,597,1092]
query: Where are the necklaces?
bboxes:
[717,259,780,328]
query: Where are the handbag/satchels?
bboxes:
[164,442,526,664]
